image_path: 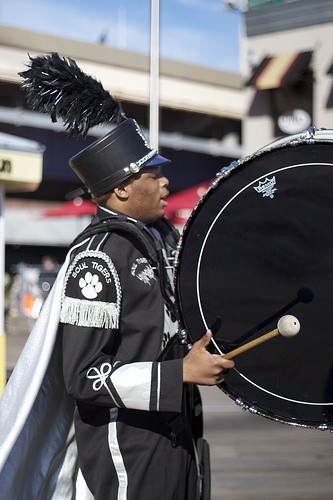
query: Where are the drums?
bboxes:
[174,129,333,431]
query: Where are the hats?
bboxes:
[18,50,170,200]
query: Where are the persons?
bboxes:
[44,118,235,500]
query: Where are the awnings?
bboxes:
[243,49,313,90]
